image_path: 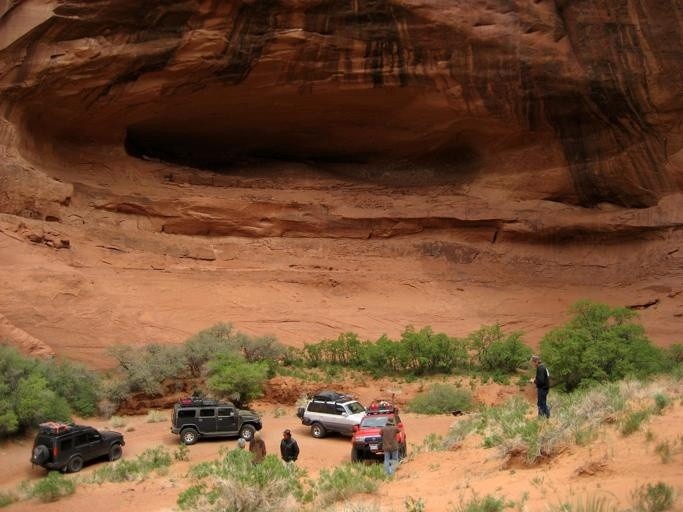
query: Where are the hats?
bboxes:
[284,429,291,436]
[387,417,395,425]
[532,356,541,363]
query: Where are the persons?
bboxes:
[248,431,266,467]
[236,438,245,449]
[280,430,298,467]
[528,355,550,418]
[379,417,401,479]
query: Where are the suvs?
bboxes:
[296,391,406,461]
[30,421,125,472]
[171,397,262,445]
